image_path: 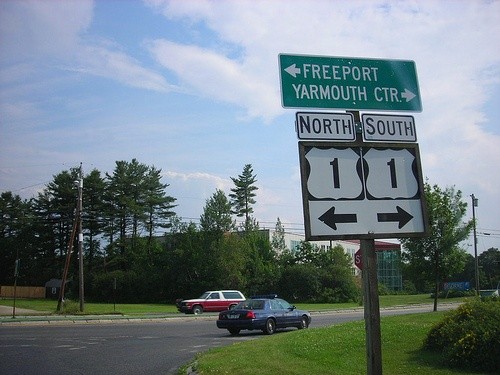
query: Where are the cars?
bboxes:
[216,294,312,335]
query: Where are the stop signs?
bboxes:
[354,248,379,271]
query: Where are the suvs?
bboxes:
[179,290,247,315]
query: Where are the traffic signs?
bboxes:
[278,54,422,114]
[295,111,432,242]
